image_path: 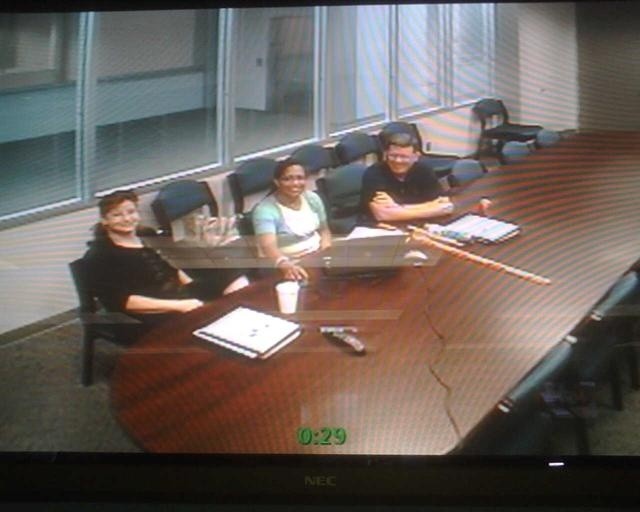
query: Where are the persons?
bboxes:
[357,132,454,226]
[252,156,331,283]
[86,190,250,340]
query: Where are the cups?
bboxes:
[276,281,300,315]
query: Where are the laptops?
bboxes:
[319,235,406,281]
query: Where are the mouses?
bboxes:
[298,278,310,288]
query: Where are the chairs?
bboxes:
[481,335,595,470]
[229,157,283,214]
[338,131,379,164]
[287,143,334,172]
[69,259,143,387]
[379,123,462,177]
[586,269,638,412]
[447,159,485,187]
[317,162,369,233]
[476,97,542,162]
[151,178,219,237]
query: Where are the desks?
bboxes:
[113,129,639,468]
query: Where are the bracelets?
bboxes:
[274,256,289,269]
